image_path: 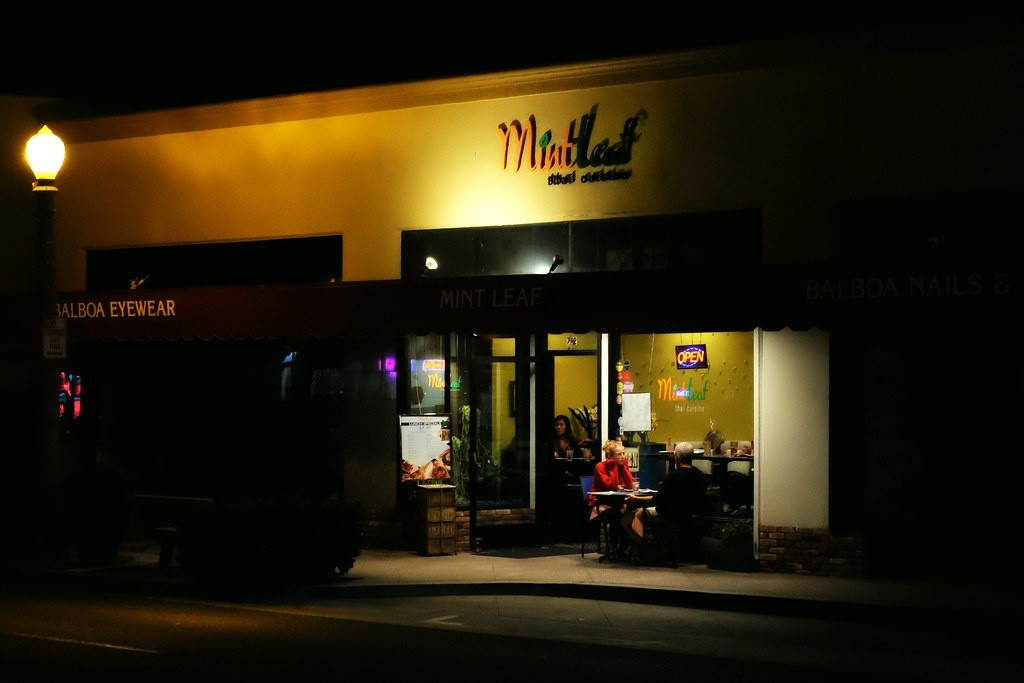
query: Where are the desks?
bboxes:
[587,489,659,566]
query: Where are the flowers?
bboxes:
[568,403,658,442]
[711,514,753,541]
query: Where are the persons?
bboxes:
[588,430,725,563]
[554,416,584,458]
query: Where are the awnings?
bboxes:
[62,269,831,336]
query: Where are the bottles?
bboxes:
[666,437,673,450]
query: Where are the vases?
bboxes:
[703,536,753,573]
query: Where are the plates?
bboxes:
[658,449,704,454]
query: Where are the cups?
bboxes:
[731,442,738,456]
[703,442,711,456]
[631,478,640,494]
[567,450,573,461]
[617,483,624,492]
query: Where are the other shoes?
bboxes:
[658,546,668,560]
[645,550,657,562]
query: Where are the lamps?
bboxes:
[419,255,440,278]
[548,255,564,273]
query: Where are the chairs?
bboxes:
[639,480,709,565]
[580,476,622,562]
